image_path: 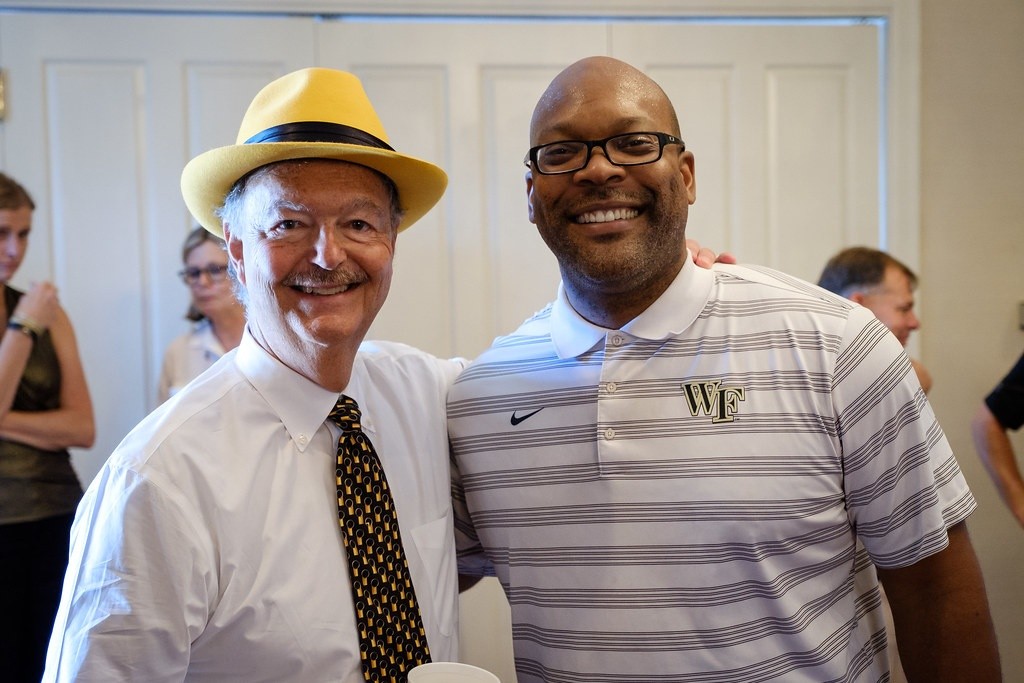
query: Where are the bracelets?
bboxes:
[7,310,45,344]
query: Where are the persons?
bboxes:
[152,227,247,408]
[815,246,933,397]
[971,346,1024,530]
[37,65,737,683]
[444,56,1004,683]
[0,171,97,683]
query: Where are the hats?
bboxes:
[181,67,448,240]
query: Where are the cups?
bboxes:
[408,662,500,683]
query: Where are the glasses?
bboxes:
[178,263,228,281]
[524,130,686,176]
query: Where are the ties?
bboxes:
[327,394,433,683]
